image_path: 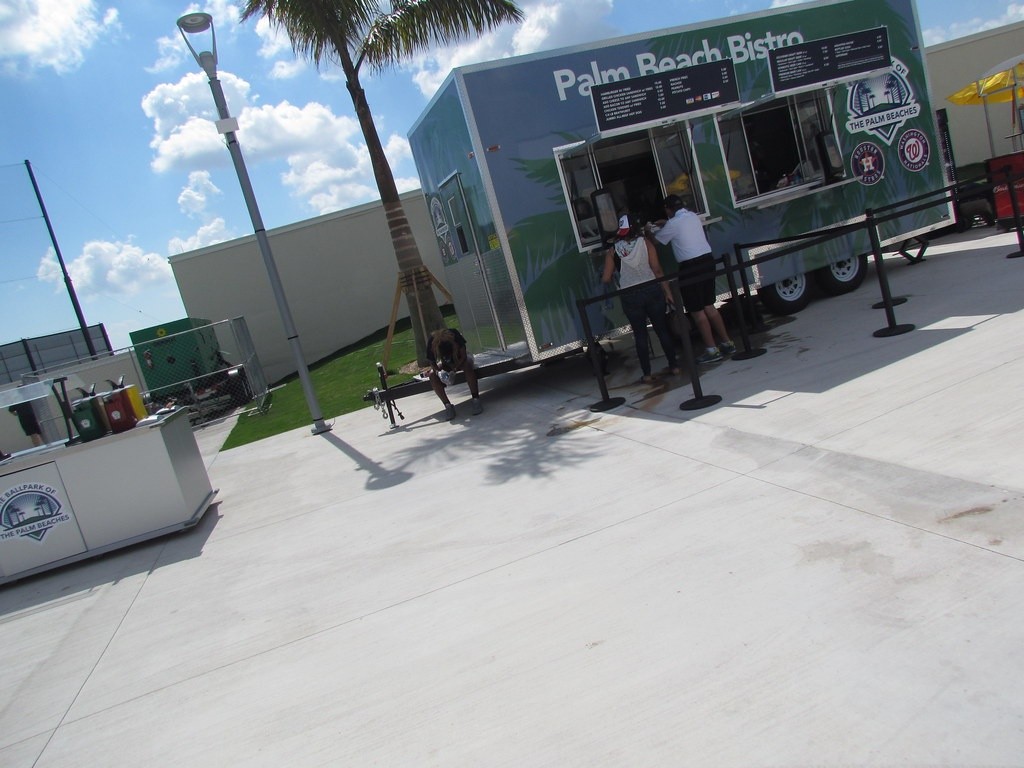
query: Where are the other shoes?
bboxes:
[472,399,482,415]
[446,404,455,421]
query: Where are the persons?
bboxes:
[644,194,737,364]
[9,384,45,447]
[426,328,483,421]
[602,213,681,384]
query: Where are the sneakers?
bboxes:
[696,347,723,363]
[716,339,737,353]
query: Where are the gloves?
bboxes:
[448,371,455,385]
[437,369,450,386]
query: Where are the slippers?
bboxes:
[663,366,679,374]
[638,376,654,383]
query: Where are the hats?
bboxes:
[658,194,682,206]
[616,214,636,236]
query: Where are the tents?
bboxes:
[945,54,1024,157]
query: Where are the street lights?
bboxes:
[176,10,333,435]
